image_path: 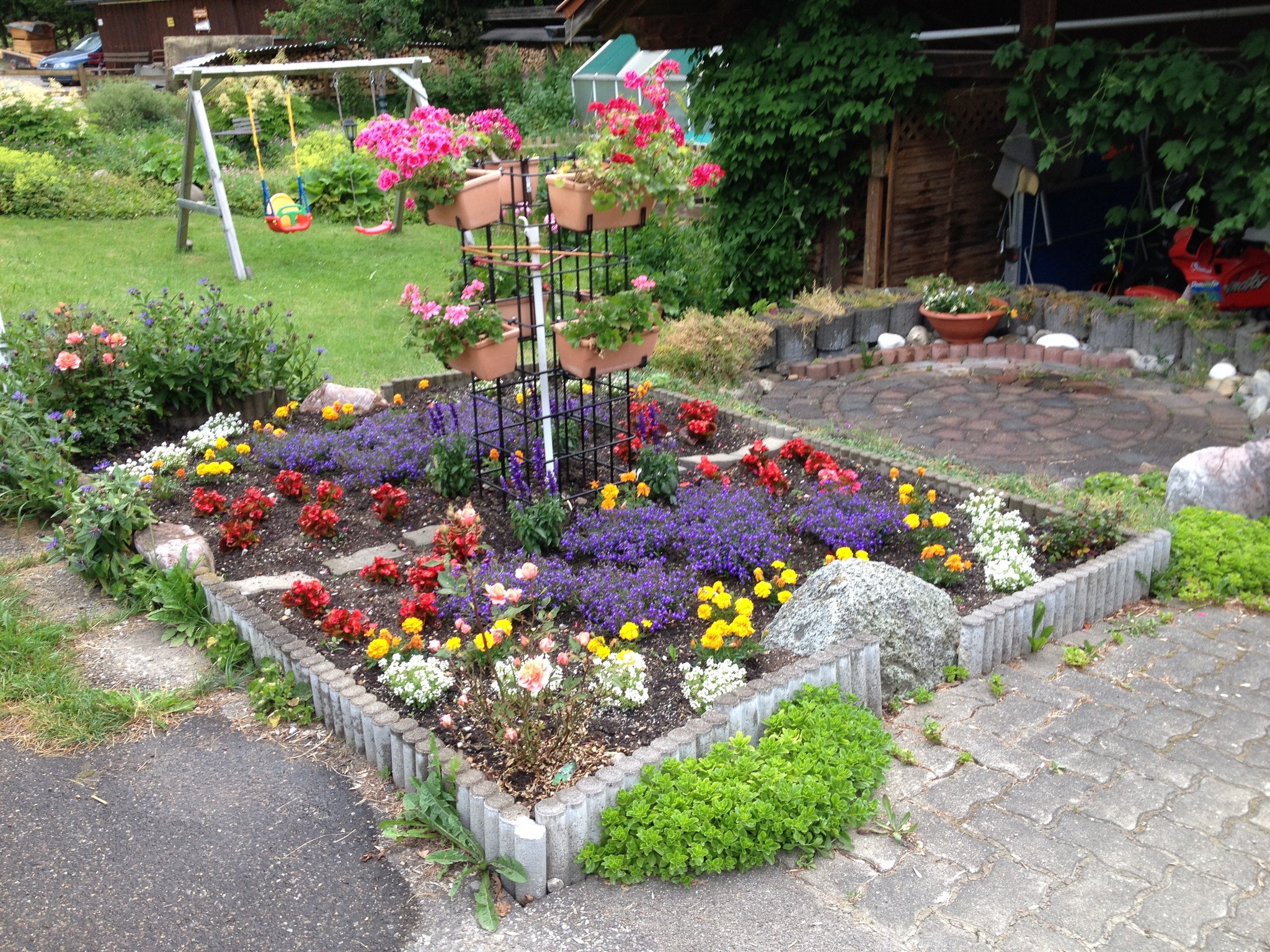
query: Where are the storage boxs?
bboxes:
[12,24,56,54]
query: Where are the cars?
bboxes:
[36,30,103,86]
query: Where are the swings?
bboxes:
[242,74,312,235]
[332,71,396,235]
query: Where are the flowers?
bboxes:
[348,61,728,363]
[919,272,1020,320]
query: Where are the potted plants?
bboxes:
[648,275,1270,389]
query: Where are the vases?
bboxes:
[438,322,523,380]
[543,172,655,232]
[470,292,550,340]
[575,289,666,326]
[411,167,502,230]
[553,320,666,379]
[921,293,1014,345]
[469,157,542,205]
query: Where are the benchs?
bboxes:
[92,51,149,74]
